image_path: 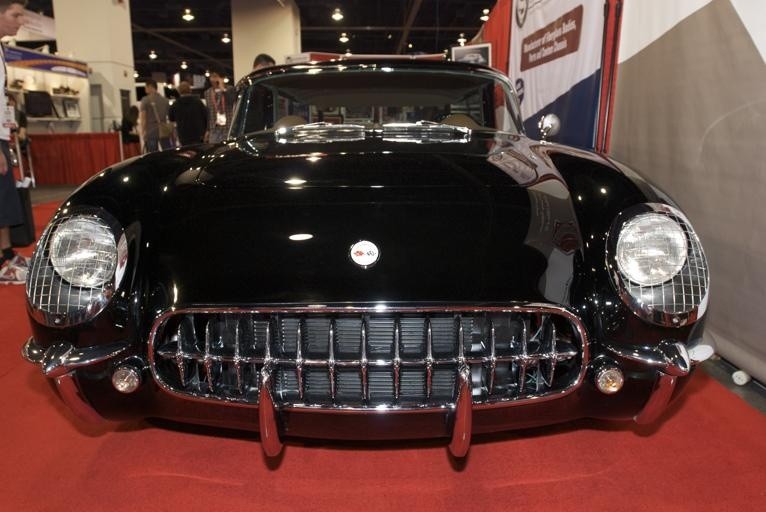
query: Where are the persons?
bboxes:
[202,64,235,144]
[0,0,34,287]
[168,81,207,145]
[244,54,276,134]
[121,105,140,144]
[5,92,29,155]
[139,80,173,152]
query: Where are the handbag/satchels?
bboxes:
[159,123,176,138]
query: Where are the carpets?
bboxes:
[0,199,766,511]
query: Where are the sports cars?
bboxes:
[21,57,717,461]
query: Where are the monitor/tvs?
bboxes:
[135,85,149,101]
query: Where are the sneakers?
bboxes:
[0,253,31,285]
